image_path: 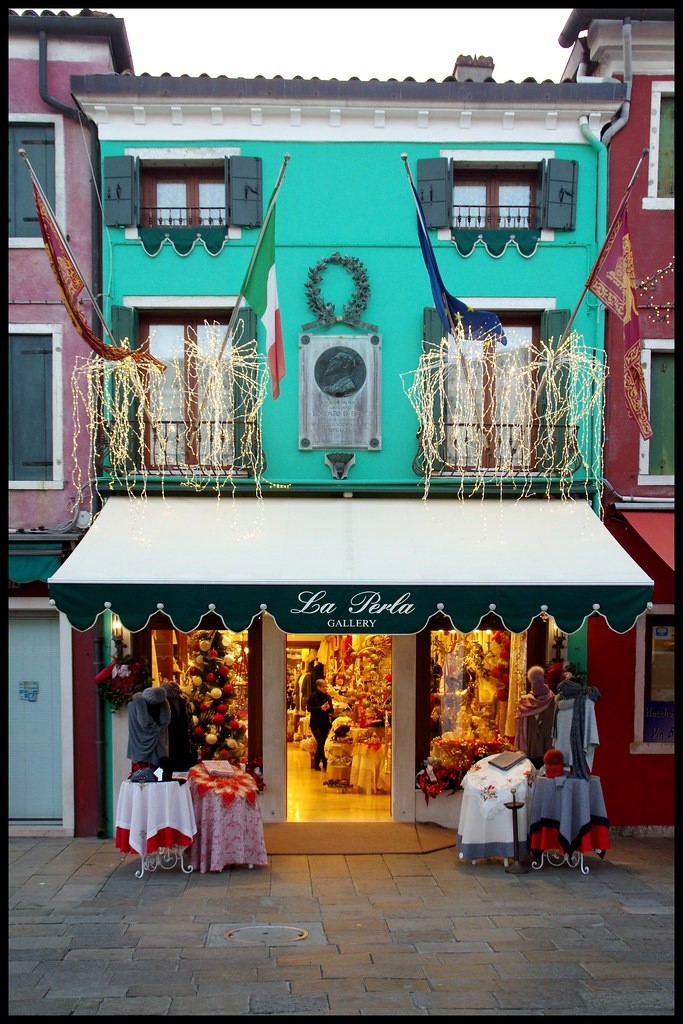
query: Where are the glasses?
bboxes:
[321,685,328,688]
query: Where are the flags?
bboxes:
[238,183,287,399]
[412,190,509,346]
[29,170,168,373]
[582,190,656,441]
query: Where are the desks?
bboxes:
[528,773,611,875]
[115,779,198,879]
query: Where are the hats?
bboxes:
[162,682,180,697]
[143,688,166,704]
[528,666,549,696]
[131,769,158,781]
[543,750,564,778]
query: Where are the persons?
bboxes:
[155,681,198,773]
[307,679,335,772]
[514,665,555,771]
[293,670,312,711]
[308,657,324,693]
[551,674,600,773]
[330,673,347,686]
[126,688,172,766]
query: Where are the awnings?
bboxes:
[43,495,653,635]
[609,501,675,602]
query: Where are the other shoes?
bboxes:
[324,758,327,767]
[315,763,321,770]
